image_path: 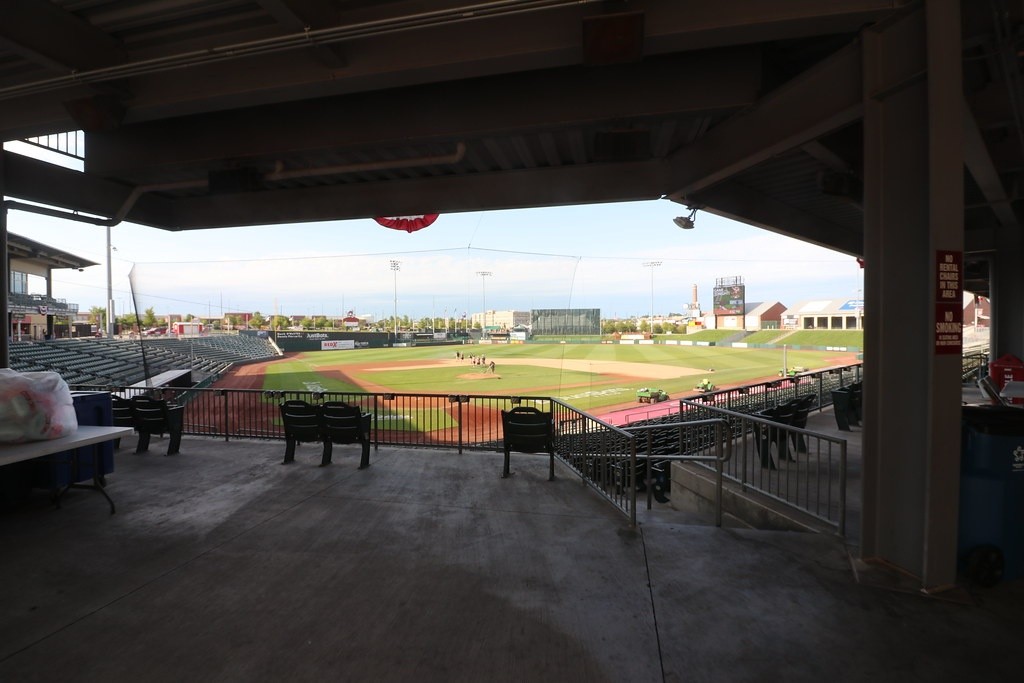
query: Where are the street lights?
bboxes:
[476,272,493,341]
[645,262,663,333]
[390,260,401,338]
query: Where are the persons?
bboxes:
[490,361,495,374]
[455,350,465,363]
[472,355,476,364]
[481,354,486,366]
[477,356,480,365]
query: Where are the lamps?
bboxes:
[673,206,703,230]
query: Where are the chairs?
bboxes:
[501,407,555,482]
[8,337,232,390]
[131,395,185,456]
[278,399,322,466]
[978,375,1024,409]
[557,396,790,500]
[112,395,134,449]
[318,401,373,470]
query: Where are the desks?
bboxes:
[999,381,1024,398]
[0,426,134,515]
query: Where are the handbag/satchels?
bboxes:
[0,369,79,444]
